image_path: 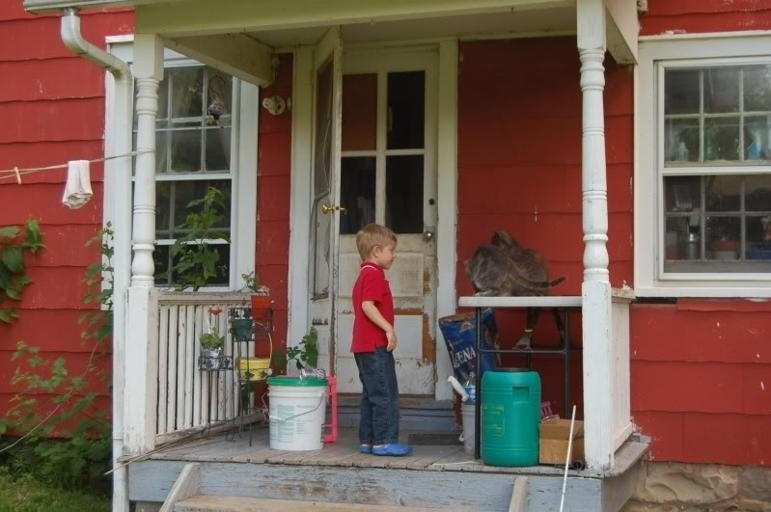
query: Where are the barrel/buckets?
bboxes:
[458,402,476,457]
[262,376,329,451]
[479,367,543,468]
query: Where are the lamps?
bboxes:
[263,93,285,117]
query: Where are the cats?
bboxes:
[463,245,566,297]
[491,228,565,349]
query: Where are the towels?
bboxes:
[59,154,95,211]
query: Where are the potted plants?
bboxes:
[239,272,272,316]
[198,329,225,370]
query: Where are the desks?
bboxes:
[458,293,585,461]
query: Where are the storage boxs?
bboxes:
[537,416,586,467]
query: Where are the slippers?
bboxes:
[373,441,412,456]
[359,443,371,454]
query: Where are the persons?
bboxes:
[351,222,413,456]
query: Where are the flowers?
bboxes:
[208,295,254,319]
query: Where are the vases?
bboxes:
[230,318,255,340]
[235,357,273,381]
